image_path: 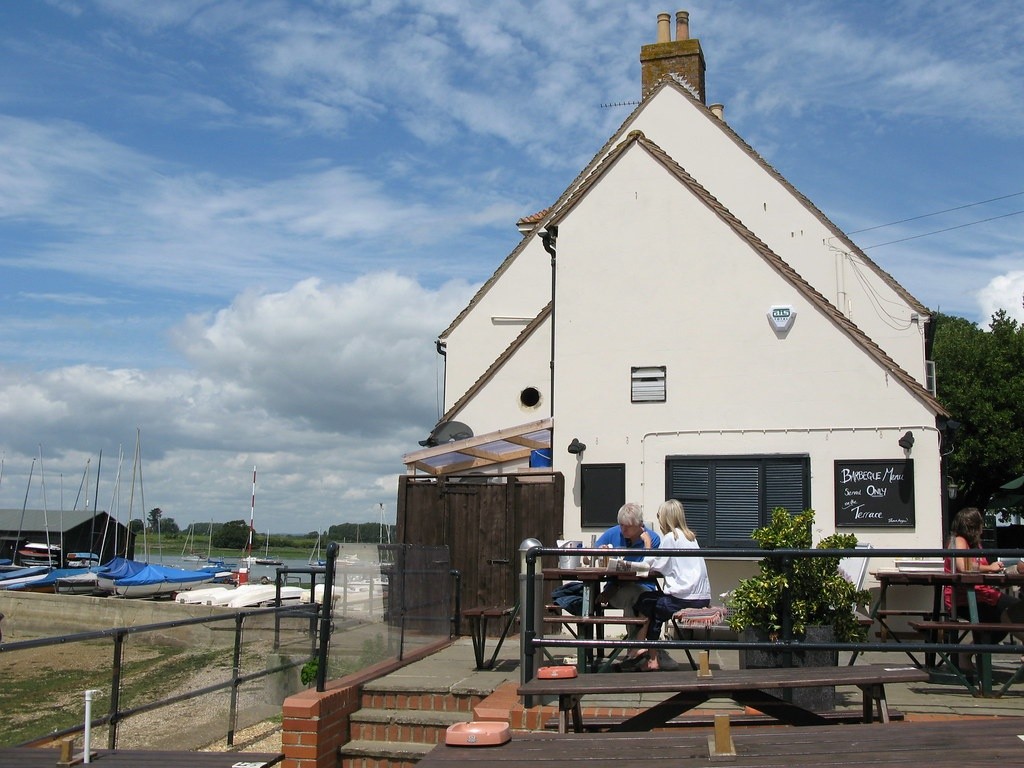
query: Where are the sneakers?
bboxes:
[657,650,679,669]
[621,655,634,670]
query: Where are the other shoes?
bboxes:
[631,649,648,661]
[639,659,660,672]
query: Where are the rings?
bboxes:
[998,564,1001,568]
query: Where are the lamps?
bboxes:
[568,438,585,454]
[898,430,915,449]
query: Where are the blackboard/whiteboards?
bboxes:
[834,458,916,528]
[580,463,626,528]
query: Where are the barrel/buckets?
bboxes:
[557,540,582,569]
[531,448,553,468]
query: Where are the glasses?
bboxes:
[657,513,659,518]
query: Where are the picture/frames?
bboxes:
[580,462,626,528]
[833,458,915,528]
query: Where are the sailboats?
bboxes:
[0,427,215,598]
[349,502,395,566]
[255,529,284,565]
[181,518,212,562]
[306,528,327,566]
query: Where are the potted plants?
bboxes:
[718,507,871,715]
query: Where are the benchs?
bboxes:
[415,718,1024,768]
[516,603,726,626]
[519,663,931,722]
[849,609,1024,631]
[462,606,514,669]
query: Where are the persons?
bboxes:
[1000,554,1024,663]
[945,507,1018,675]
[623,499,711,672]
[582,503,660,661]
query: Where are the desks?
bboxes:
[874,570,1024,697]
[542,567,664,674]
